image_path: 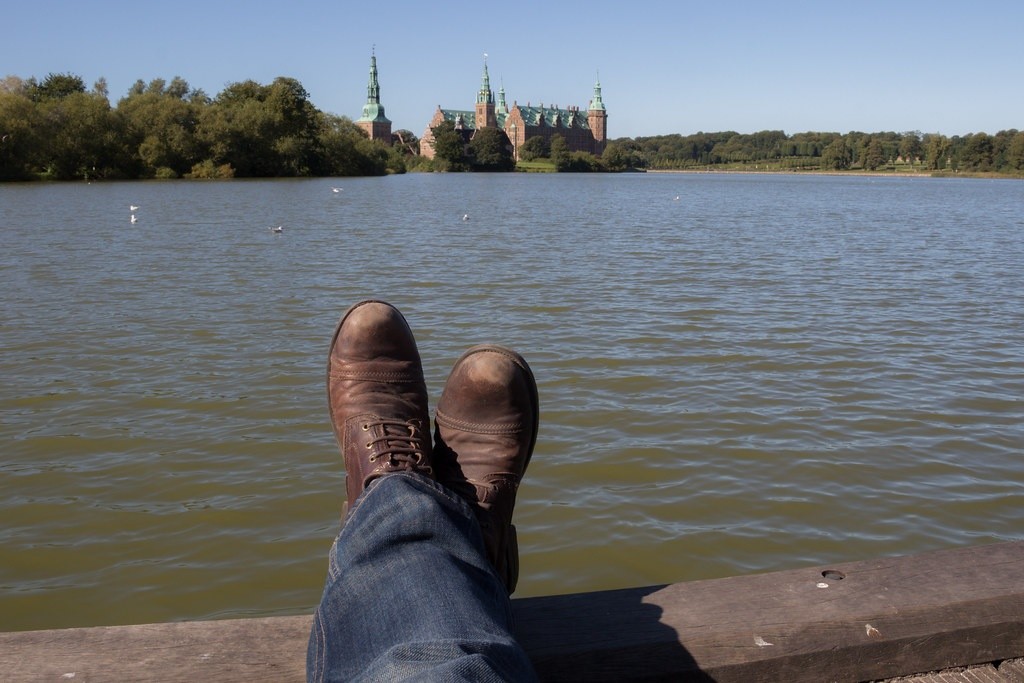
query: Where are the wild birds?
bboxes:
[333,188,344,192]
[130,206,139,210]
[677,195,679,199]
[268,226,284,233]
[463,215,470,221]
[131,215,138,223]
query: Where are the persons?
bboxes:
[305,301,540,683]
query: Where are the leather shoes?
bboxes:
[435,345,539,598]
[325,300,434,542]
